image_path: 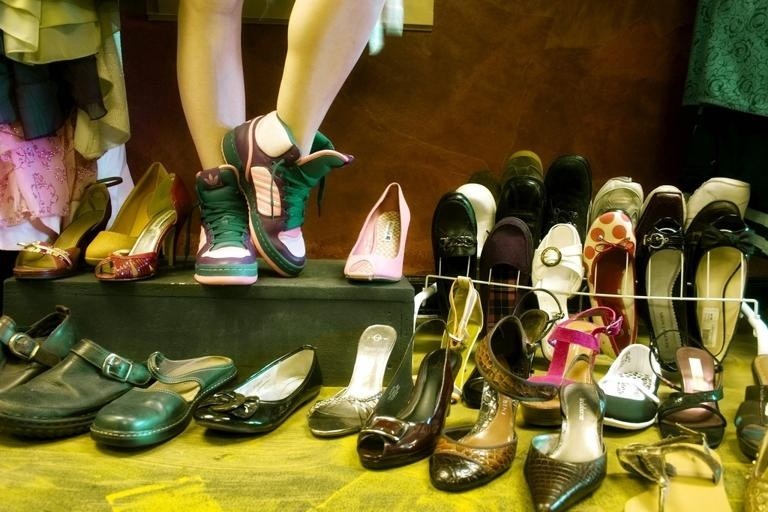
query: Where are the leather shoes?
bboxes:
[430,149,768,449]
[192,343,322,433]
[0,337,157,443]
[90,351,238,448]
[343,181,411,282]
[0,305,73,395]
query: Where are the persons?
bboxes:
[173,0,390,287]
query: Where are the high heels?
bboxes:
[429,314,559,492]
[523,381,610,512]
[12,176,123,280]
[308,325,398,437]
[83,161,192,283]
[355,320,462,468]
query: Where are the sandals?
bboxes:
[615,431,732,511]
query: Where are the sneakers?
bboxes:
[221,111,354,278]
[194,164,259,286]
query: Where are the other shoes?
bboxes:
[743,432,768,512]
